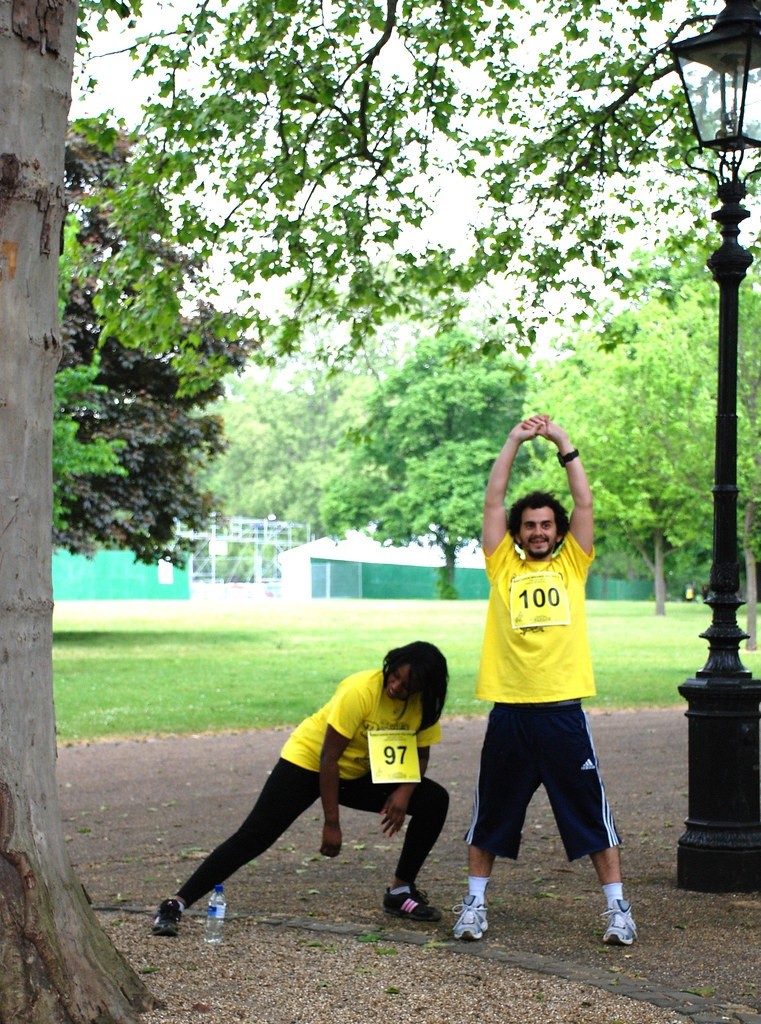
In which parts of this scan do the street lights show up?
[669,0,761,893]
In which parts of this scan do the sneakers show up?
[152,897,183,935]
[383,883,441,921]
[600,899,638,946]
[452,895,488,940]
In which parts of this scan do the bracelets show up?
[556,448,579,468]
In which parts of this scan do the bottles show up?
[204,884,227,942]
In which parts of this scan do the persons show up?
[150,639,454,936]
[449,411,640,948]
